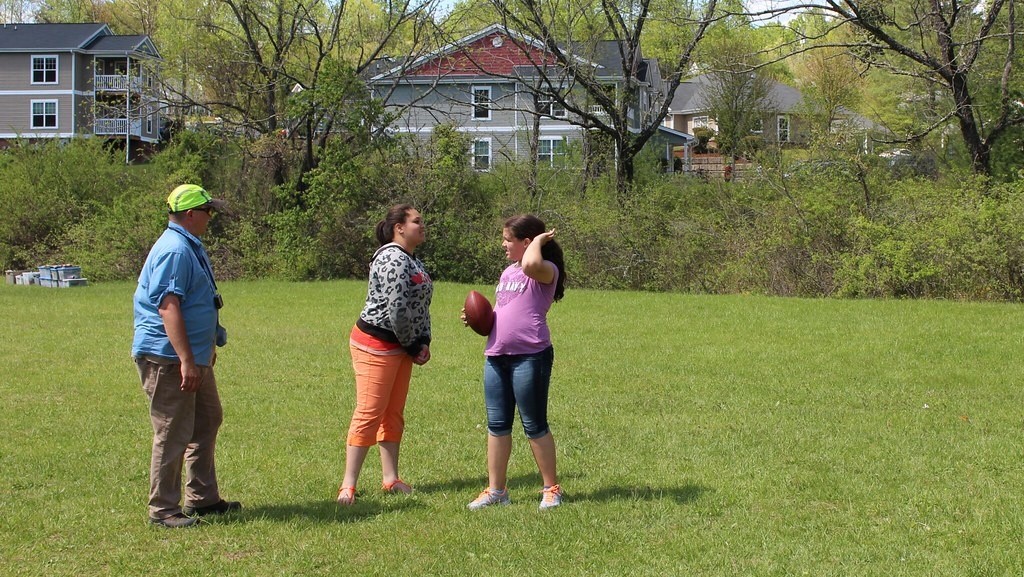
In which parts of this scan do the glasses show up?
[192,205,215,216]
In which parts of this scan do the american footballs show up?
[464,290,493,335]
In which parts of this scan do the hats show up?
[167,183,224,214]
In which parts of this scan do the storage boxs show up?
[21,271,39,285]
[38,276,51,288]
[31,271,40,285]
[49,267,59,280]
[14,274,24,284]
[50,279,58,287]
[57,277,87,287]
[4,269,30,285]
[56,267,80,279]
[37,264,61,279]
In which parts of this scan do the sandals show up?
[336,484,357,506]
[381,477,417,495]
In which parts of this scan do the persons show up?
[460,215,566,510]
[131,183,240,528]
[334,204,433,507]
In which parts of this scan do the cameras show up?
[215,293,223,309]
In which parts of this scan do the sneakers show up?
[148,511,200,528]
[465,485,510,512]
[537,482,563,509]
[183,498,242,517]
